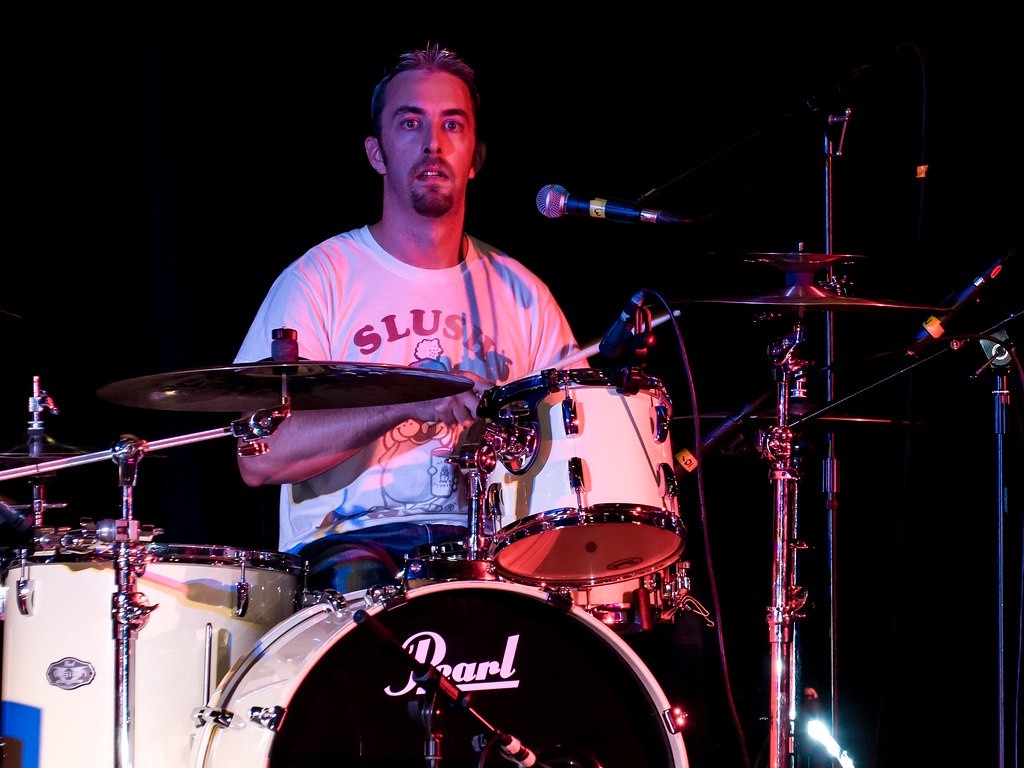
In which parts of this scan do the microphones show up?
[536,185,681,225]
[499,731,550,768]
[906,259,1005,356]
[673,392,778,481]
[599,289,645,358]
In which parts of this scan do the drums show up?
[191,578,691,768]
[482,366,691,590]
[403,533,677,645]
[0,534,310,768]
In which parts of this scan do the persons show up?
[228,46,593,591]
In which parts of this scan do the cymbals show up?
[669,394,927,428]
[91,353,476,414]
[647,249,959,315]
